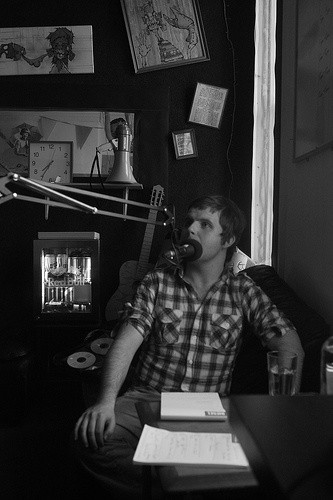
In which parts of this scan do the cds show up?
[66,352,96,369]
[90,338,114,355]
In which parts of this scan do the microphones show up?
[164,244,196,259]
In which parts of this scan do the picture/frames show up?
[0,25,94,76]
[115,0,211,76]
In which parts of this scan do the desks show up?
[134,394,333,500]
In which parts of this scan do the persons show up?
[73,194,305,500]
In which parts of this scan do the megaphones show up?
[95,125,138,185]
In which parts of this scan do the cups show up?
[268,351,297,396]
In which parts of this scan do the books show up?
[132,424,249,469]
[160,392,227,422]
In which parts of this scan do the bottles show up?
[321,336,333,397]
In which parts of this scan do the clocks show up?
[29,141,73,183]
